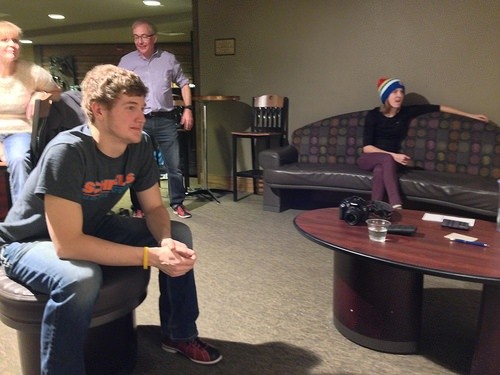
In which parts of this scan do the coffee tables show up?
[293,207,500,375]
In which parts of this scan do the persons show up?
[117,20,194,218]
[0,21,62,205]
[0,64,223,375]
[357,79,488,209]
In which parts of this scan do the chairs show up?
[229,95,287,201]
[0,93,52,205]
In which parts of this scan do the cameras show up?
[340,197,372,226]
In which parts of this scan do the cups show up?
[365,218,391,242]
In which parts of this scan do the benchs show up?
[256,109,500,223]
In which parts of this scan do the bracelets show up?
[184,105,193,110]
[143,247,149,269]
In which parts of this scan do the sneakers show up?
[173,204,192,218]
[132,209,143,219]
[161,335,223,364]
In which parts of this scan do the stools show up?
[0,261,151,375]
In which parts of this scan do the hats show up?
[377,77,404,104]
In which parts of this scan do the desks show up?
[168,95,241,204]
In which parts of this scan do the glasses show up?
[133,33,155,41]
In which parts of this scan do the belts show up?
[144,111,174,119]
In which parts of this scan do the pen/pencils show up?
[450,238,487,247]
